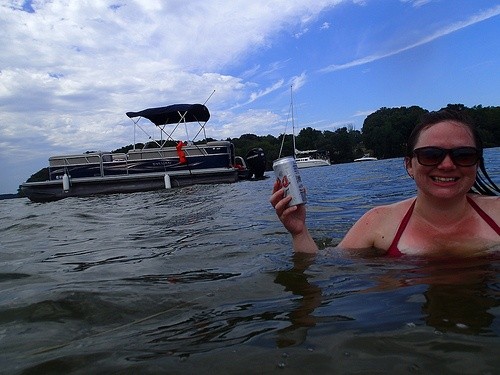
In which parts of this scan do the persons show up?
[269,115,500,258]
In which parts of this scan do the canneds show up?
[272,156,307,207]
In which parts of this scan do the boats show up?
[354,153,378,161]
[279,85,331,167]
[20,102,265,202]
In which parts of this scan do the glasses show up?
[410,146,481,167]
